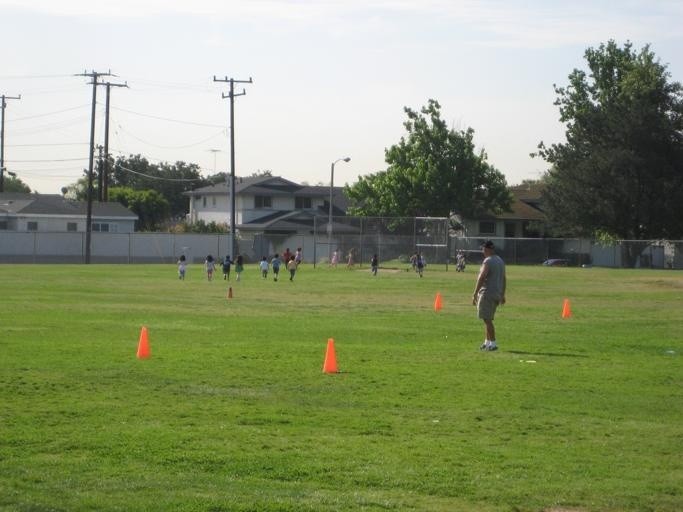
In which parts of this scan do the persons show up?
[218,254,233,281]
[232,256,244,281]
[176,255,186,280]
[203,255,216,281]
[294,247,304,265]
[286,256,296,281]
[269,252,281,282]
[259,256,268,278]
[330,247,466,279]
[282,248,291,269]
[471,239,507,351]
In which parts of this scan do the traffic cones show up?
[226,286,234,299]
[433,289,444,311]
[319,336,342,374]
[561,297,573,320]
[132,325,155,361]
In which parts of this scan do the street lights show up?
[327,156,353,262]
[210,148,221,174]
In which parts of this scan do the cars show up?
[540,256,576,267]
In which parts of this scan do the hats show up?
[480,240,494,247]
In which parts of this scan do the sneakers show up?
[478,343,498,351]
